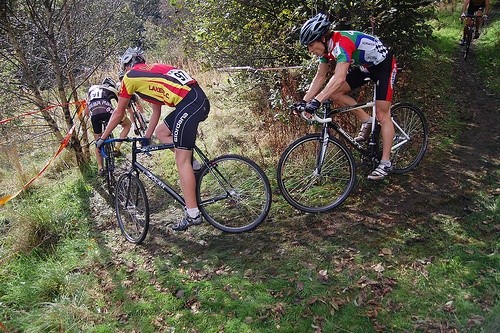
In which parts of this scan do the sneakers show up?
[97,167,105,176]
[112,149,122,157]
[474,31,480,39]
[459,37,466,46]
[192,166,210,176]
[367,161,394,182]
[171,211,204,231]
[354,117,379,143]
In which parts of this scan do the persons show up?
[460,0,490,45]
[289,13,399,179]
[88,46,211,231]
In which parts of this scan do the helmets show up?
[102,77,116,88]
[120,46,148,71]
[118,70,126,80]
[299,13,331,48]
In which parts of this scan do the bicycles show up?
[128,98,148,137]
[100,137,272,244]
[277,76,428,213]
[100,120,116,188]
[461,14,485,60]
